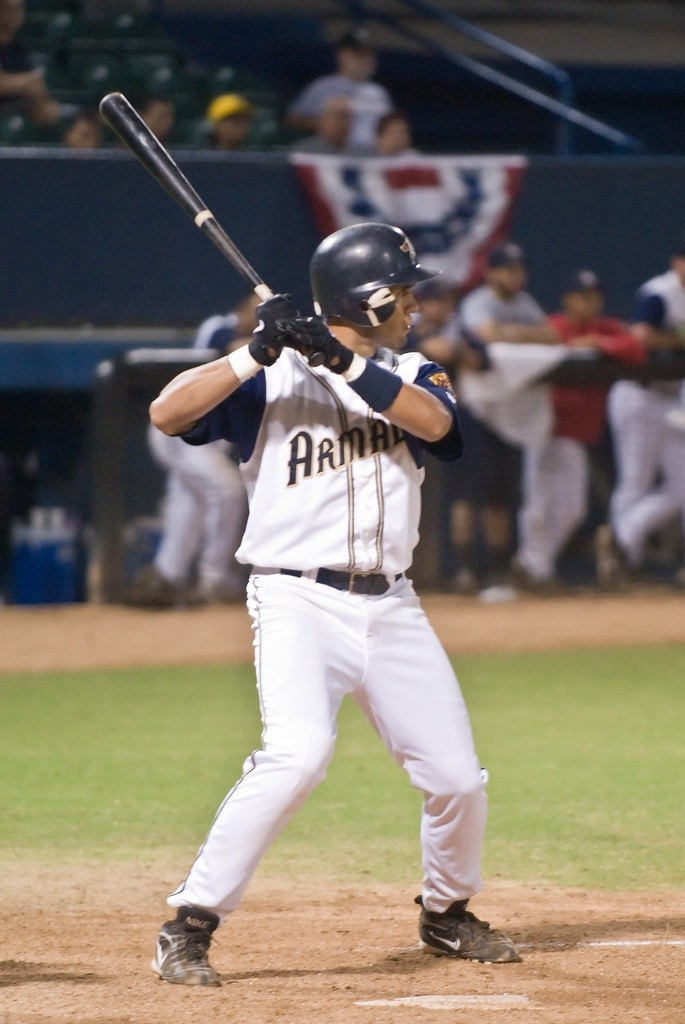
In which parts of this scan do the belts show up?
[282,568,402,594]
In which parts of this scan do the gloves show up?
[248,293,298,367]
[274,316,354,374]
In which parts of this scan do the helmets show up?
[309,221,441,327]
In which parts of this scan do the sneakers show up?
[415,893,521,964]
[151,906,220,987]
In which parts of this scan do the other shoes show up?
[595,525,630,594]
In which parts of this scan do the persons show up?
[149,221,523,987]
[448,240,685,593]
[140,91,177,138]
[60,112,96,149]
[0,0,60,122]
[150,288,265,603]
[403,280,491,381]
[285,33,417,156]
[208,94,253,151]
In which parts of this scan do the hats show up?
[565,272,598,294]
[486,242,524,269]
[208,94,252,122]
[340,26,376,59]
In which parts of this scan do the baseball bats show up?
[98,91,327,368]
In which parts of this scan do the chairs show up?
[0,0,314,152]
